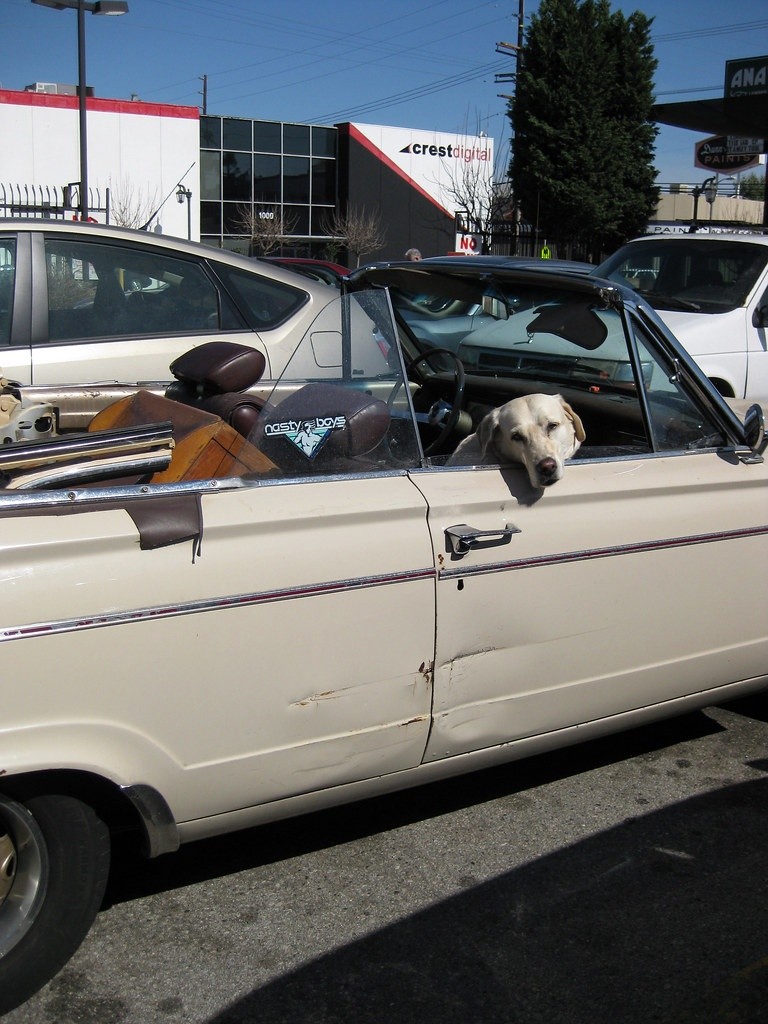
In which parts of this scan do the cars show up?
[256,254,599,300]
[0,256,768,1016]
[457,233,768,401]
[0,219,497,387]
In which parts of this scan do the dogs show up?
[445,392,586,489]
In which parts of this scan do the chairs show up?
[261,383,395,478]
[165,341,274,438]
[668,270,727,294]
[81,271,130,338]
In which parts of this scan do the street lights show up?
[689,177,716,235]
[31,0,129,285]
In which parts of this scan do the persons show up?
[405,248,422,261]
